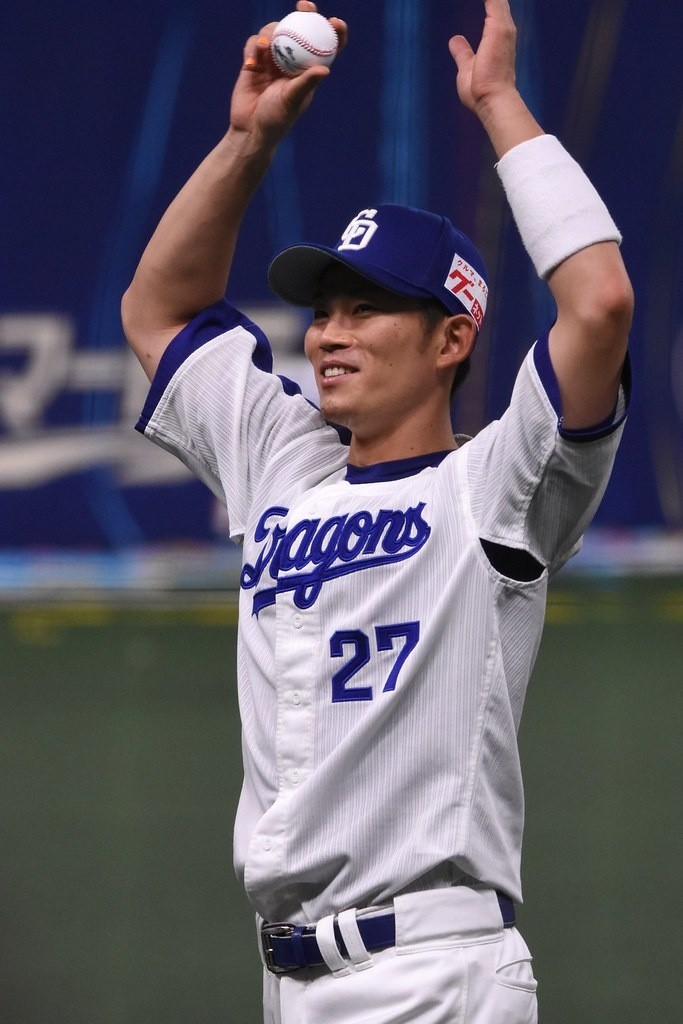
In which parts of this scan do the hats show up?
[268,202,491,339]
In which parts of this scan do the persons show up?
[118,0,636,1024]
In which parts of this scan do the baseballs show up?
[266,7,342,79]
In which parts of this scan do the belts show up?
[258,887,517,973]
